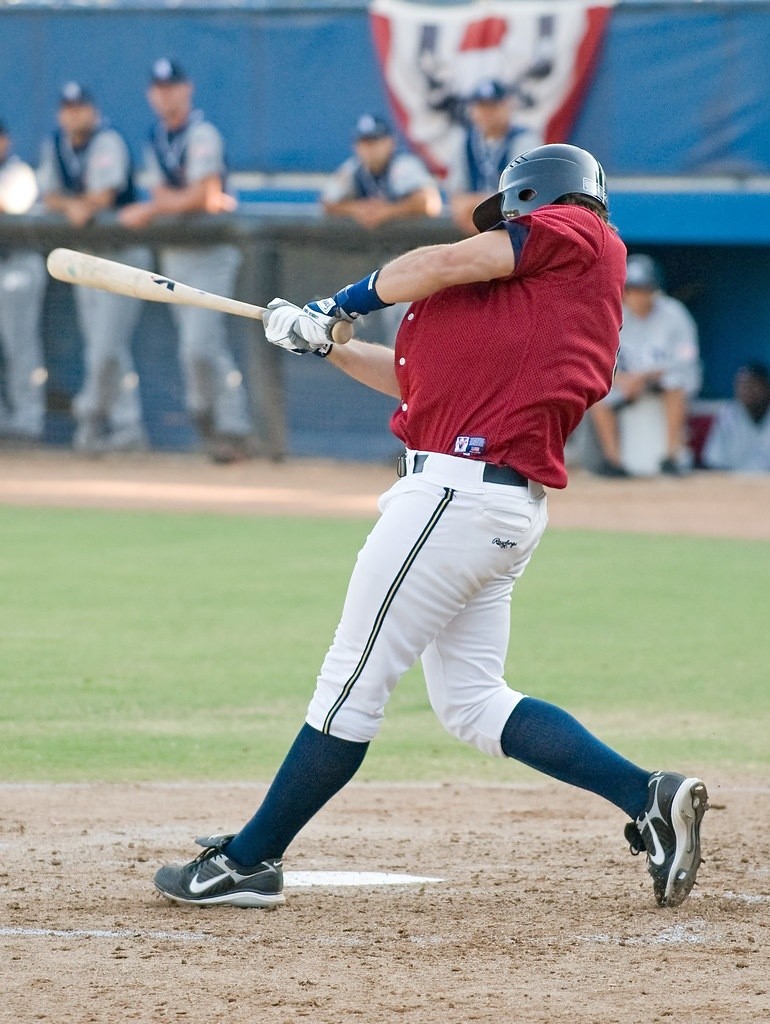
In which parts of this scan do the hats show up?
[57,83,88,106]
[148,60,182,83]
[353,115,388,141]
[621,255,658,286]
[471,81,504,103]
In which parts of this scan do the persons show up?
[118,53,250,459]
[156,143,710,908]
[320,114,442,338]
[591,251,706,478]
[33,80,154,456]
[701,363,770,469]
[0,122,50,445]
[443,82,546,236]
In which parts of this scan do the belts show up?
[397,453,529,487]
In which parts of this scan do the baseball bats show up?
[45,247,356,345]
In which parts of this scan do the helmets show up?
[471,143,610,234]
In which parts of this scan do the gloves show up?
[261,295,337,358]
[304,282,360,328]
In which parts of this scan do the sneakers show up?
[154,833,287,909]
[625,771,709,910]
